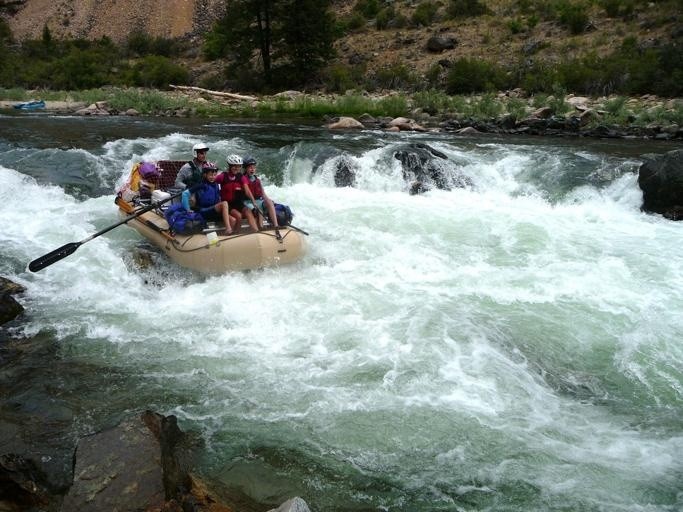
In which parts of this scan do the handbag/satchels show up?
[275,204,292,225]
[165,203,208,235]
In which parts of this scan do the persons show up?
[181,162,237,236]
[240,158,278,231]
[215,153,259,234]
[175,143,216,209]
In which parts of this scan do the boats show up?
[13,101,45,111]
[114,188,310,274]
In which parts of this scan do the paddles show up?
[29,190,181,272]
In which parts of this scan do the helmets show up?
[192,144,209,158]
[203,162,218,171]
[243,157,256,165]
[227,155,243,165]
